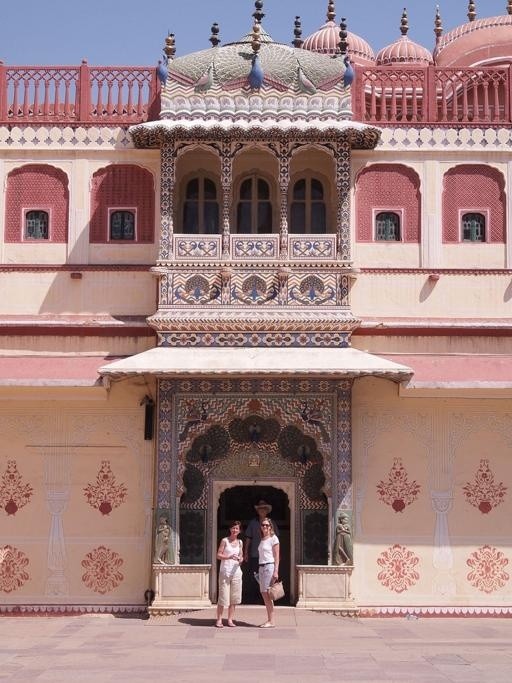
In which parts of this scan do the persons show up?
[256,517,282,628]
[241,499,281,603]
[214,520,244,628]
[155,518,172,564]
[336,512,353,566]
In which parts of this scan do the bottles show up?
[254,572,261,584]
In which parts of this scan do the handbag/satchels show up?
[268,581,285,601]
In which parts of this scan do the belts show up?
[259,562,275,566]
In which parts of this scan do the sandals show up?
[216,623,237,627]
[260,625,276,628]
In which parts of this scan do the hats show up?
[254,500,273,513]
